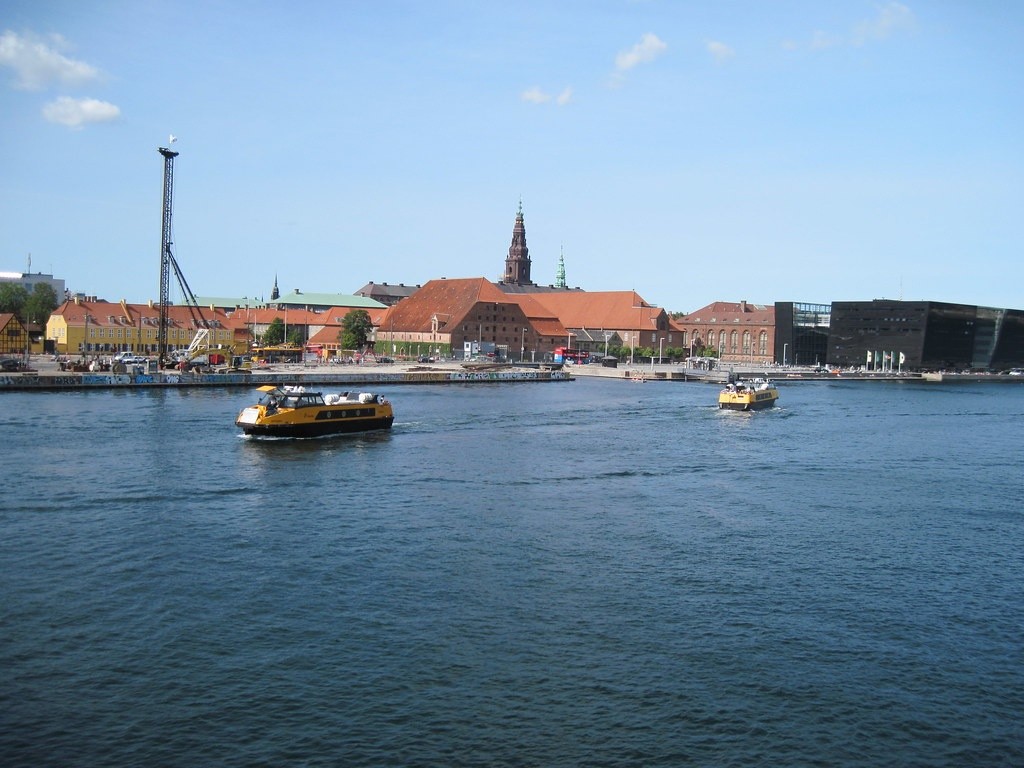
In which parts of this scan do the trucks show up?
[113,352,150,363]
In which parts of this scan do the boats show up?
[719,378,779,411]
[235,386,395,437]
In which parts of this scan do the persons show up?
[727,382,734,392]
[736,380,744,386]
[348,355,352,365]
[356,352,361,363]
[749,379,755,393]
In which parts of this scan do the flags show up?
[892,352,896,363]
[876,351,880,362]
[900,352,905,364]
[868,351,872,362]
[883,352,888,362]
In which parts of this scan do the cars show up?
[0,359,21,371]
[1009,368,1024,376]
[463,356,476,361]
[470,357,487,362]
[123,356,144,364]
[493,357,506,363]
[487,351,496,356]
[574,358,589,364]
[417,356,435,364]
[379,358,394,363]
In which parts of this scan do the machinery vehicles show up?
[184,346,252,374]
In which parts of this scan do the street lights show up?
[25,311,36,365]
[242,297,249,354]
[719,341,723,359]
[521,328,527,362]
[783,344,788,366]
[631,337,637,363]
[691,340,693,357]
[391,302,396,359]
[605,335,610,357]
[83,313,87,365]
[282,302,287,343]
[568,333,573,349]
[660,338,665,364]
[751,342,755,364]
